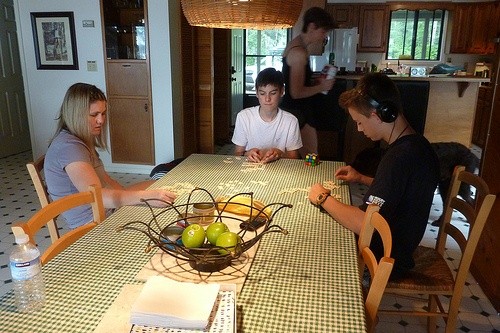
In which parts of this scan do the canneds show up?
[161,202,215,249]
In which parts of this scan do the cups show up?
[356,66,361,72]
[340,67,346,72]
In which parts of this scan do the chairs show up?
[25,153,63,245]
[358,204,394,333]
[11,184,106,268]
[363,164,496,333]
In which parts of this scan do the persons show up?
[309,71,440,263]
[44,83,176,230]
[232,68,303,164]
[283,7,336,158]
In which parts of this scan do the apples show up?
[205,222,230,244]
[216,232,242,255]
[182,224,205,248]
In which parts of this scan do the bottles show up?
[104,0,144,25]
[8,233,46,313]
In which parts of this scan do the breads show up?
[217,197,272,216]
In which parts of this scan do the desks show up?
[0,154,369,333]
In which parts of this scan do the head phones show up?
[358,90,399,123]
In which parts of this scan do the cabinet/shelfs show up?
[445,2,500,55]
[325,2,390,53]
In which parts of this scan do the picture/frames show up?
[30,11,79,71]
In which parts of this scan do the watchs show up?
[315,192,330,207]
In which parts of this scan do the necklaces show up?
[396,123,411,140]
